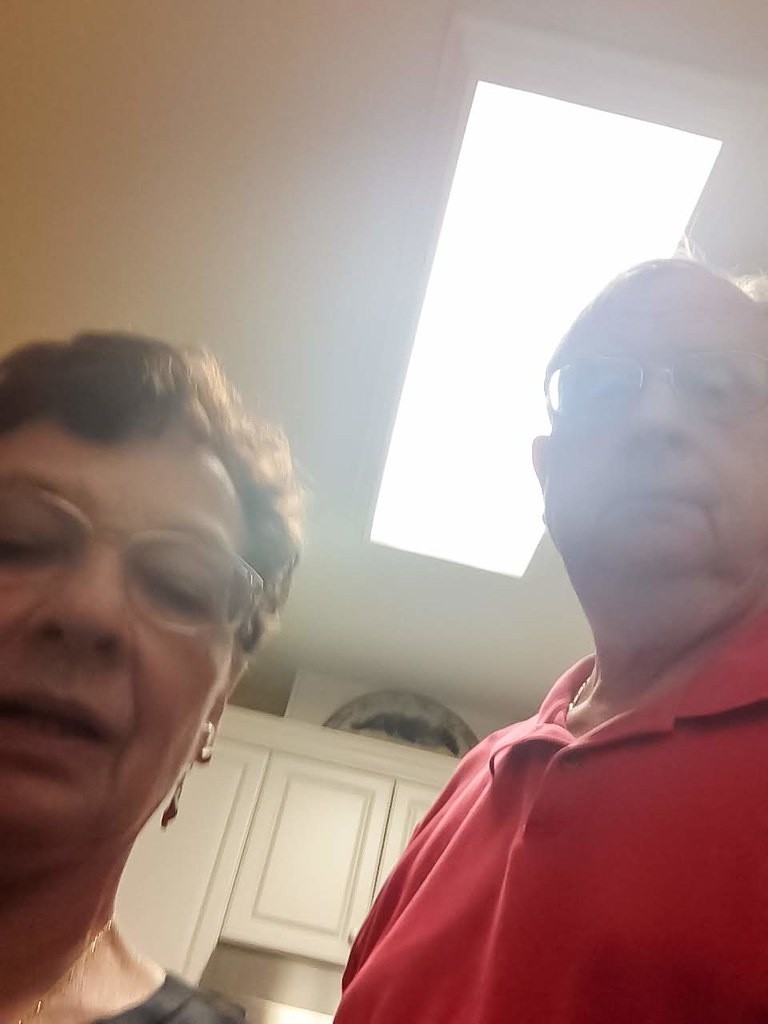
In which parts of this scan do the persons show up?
[0,329,302,1024]
[332,260,768,1024]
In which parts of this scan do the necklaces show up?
[18,911,117,1024]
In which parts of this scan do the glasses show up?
[540,352,768,427]
[0,478,263,640]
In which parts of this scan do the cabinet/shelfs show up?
[117,697,472,1024]
